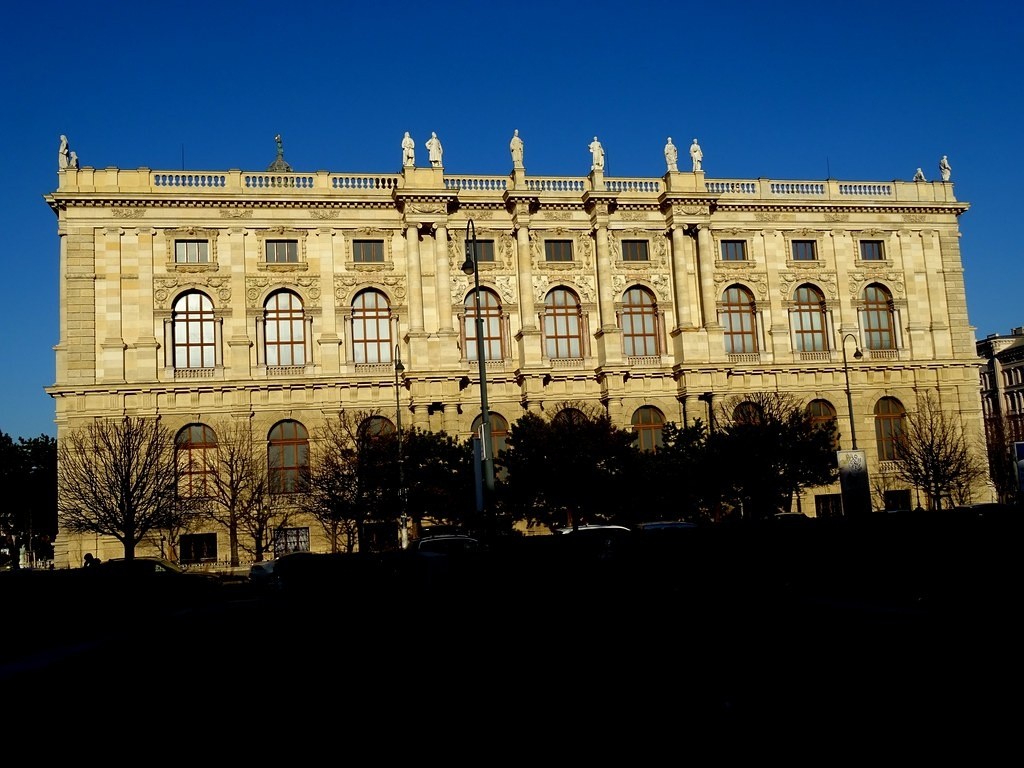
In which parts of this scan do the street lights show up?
[842,334,862,450]
[462,219,494,490]
[395,344,408,549]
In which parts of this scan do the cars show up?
[91,509,909,623]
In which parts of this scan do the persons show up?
[84,553,101,569]
